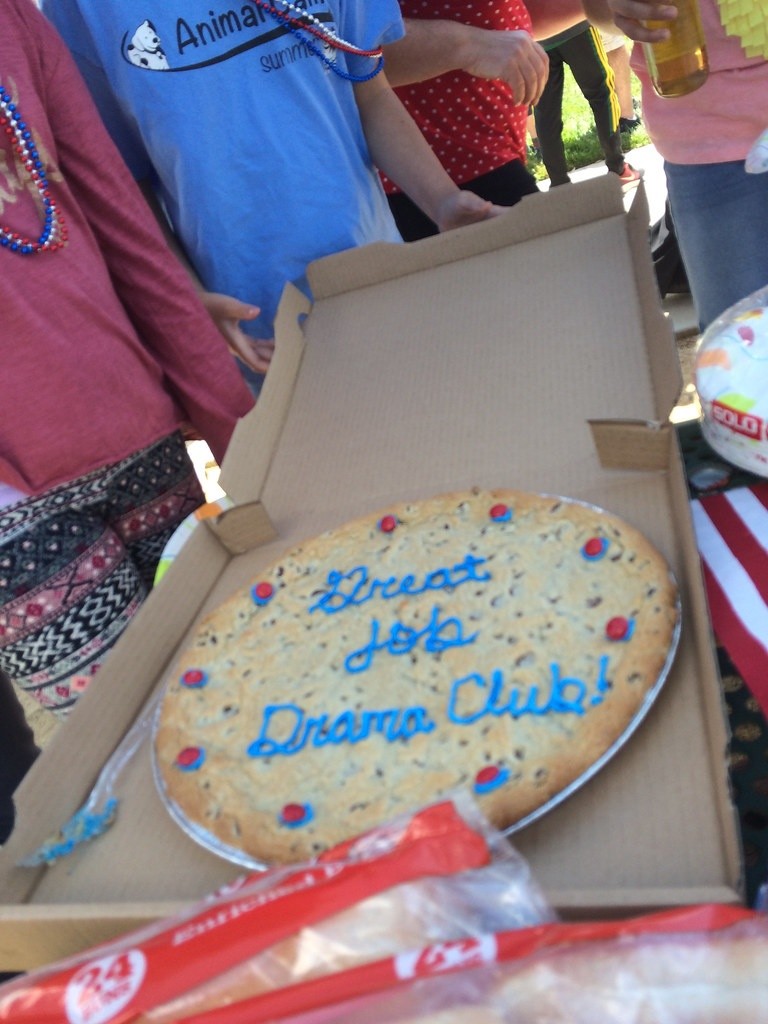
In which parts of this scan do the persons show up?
[0,0,258,726]
[377,0,549,243]
[522,0,645,192]
[607,0,768,337]
[41,0,514,404]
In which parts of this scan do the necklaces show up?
[0,85,69,254]
[255,0,384,83]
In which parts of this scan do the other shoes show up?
[615,116,642,133]
[533,146,544,161]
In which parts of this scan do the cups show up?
[639,0,710,99]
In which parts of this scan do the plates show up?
[147,502,683,877]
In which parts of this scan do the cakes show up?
[152,485,678,870]
[697,301,768,480]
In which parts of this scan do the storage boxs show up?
[0,167,752,978]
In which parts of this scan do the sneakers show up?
[619,161,646,193]
